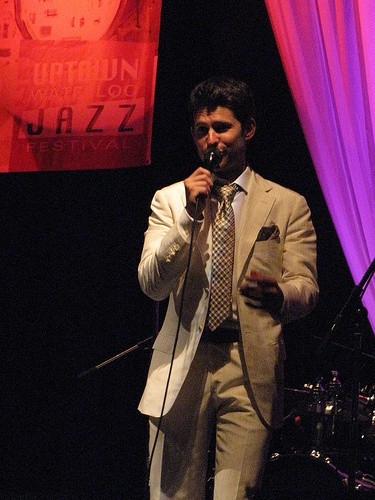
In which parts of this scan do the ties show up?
[205,184,237,331]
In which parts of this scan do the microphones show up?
[198,149,219,201]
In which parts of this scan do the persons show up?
[137,77,317,498]
